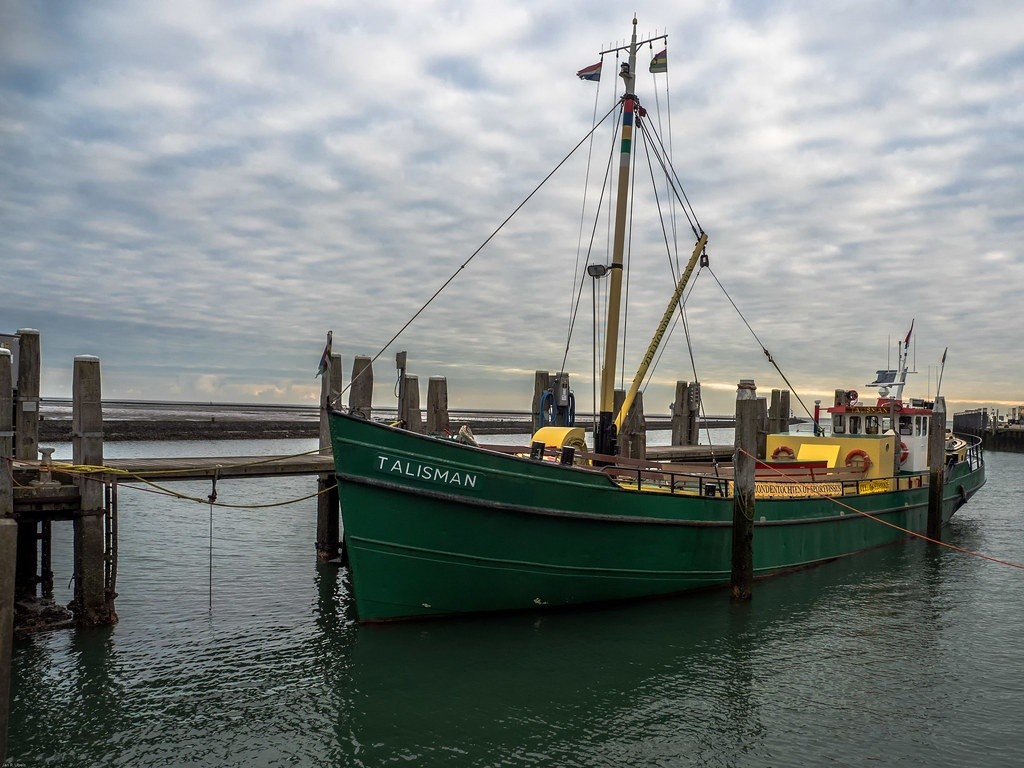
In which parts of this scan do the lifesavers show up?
[900,441,908,462]
[845,449,871,473]
[772,446,794,459]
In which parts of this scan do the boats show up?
[320,13,985,625]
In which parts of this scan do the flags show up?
[576,62,602,81]
[315,331,334,379]
[942,347,948,363]
[650,49,667,72]
[904,319,914,346]
